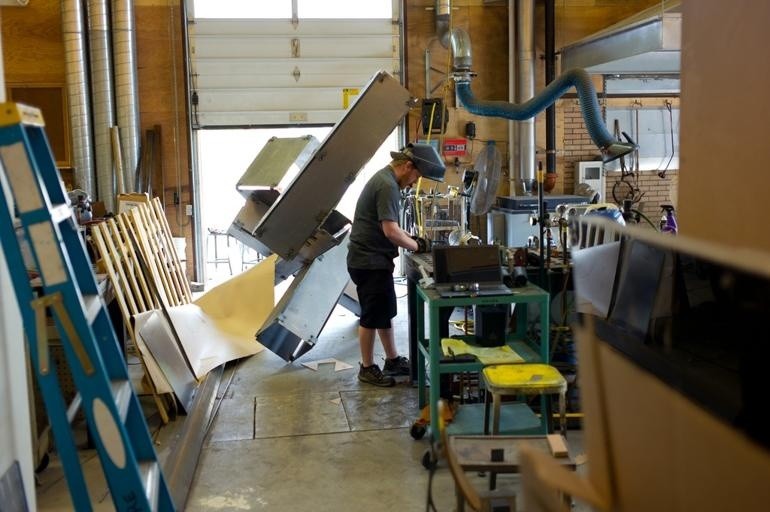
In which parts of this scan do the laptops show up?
[432,245,514,299]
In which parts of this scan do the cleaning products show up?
[660,203,678,234]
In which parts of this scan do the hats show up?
[389,146,417,162]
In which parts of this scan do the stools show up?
[481,362,569,493]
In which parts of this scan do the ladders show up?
[0,102,176,512]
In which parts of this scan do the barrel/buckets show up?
[473,304,507,346]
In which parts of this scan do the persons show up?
[345,147,432,388]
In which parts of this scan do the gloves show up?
[410,235,433,254]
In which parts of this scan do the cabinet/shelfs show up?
[413,283,550,464]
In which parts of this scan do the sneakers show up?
[381,357,413,377]
[358,361,397,389]
